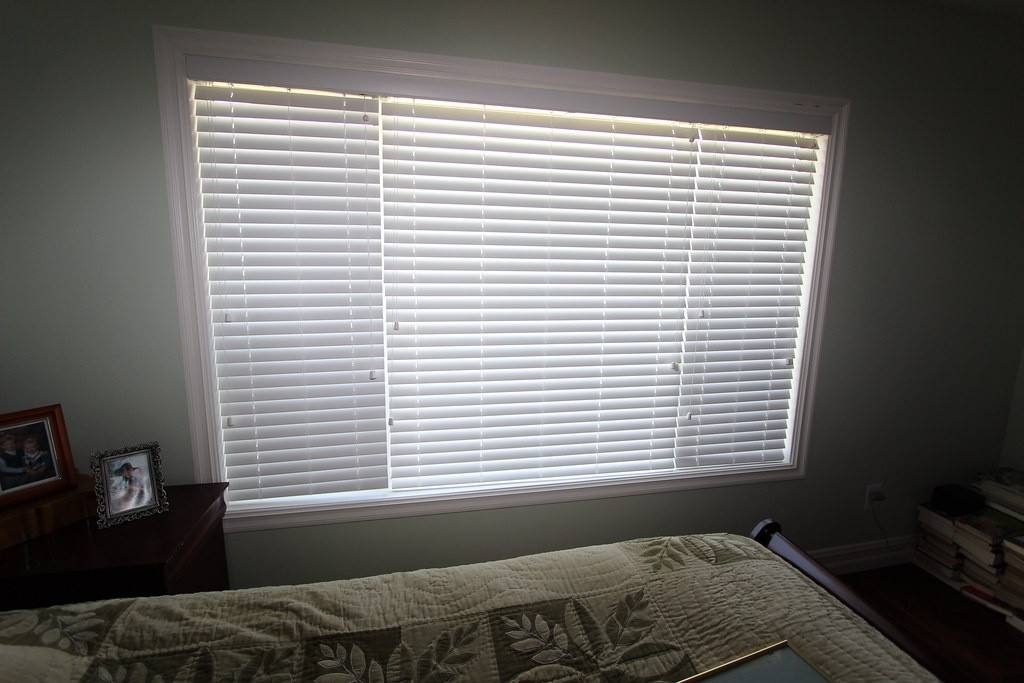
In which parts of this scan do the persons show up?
[110,463,146,514]
[0,436,52,491]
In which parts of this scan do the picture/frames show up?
[89,441,171,530]
[0,403,79,511]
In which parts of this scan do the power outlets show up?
[864,484,881,512]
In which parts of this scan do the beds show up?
[0,518,955,683]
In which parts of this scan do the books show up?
[914,466,1024,635]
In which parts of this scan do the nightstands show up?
[0,482,228,612]
